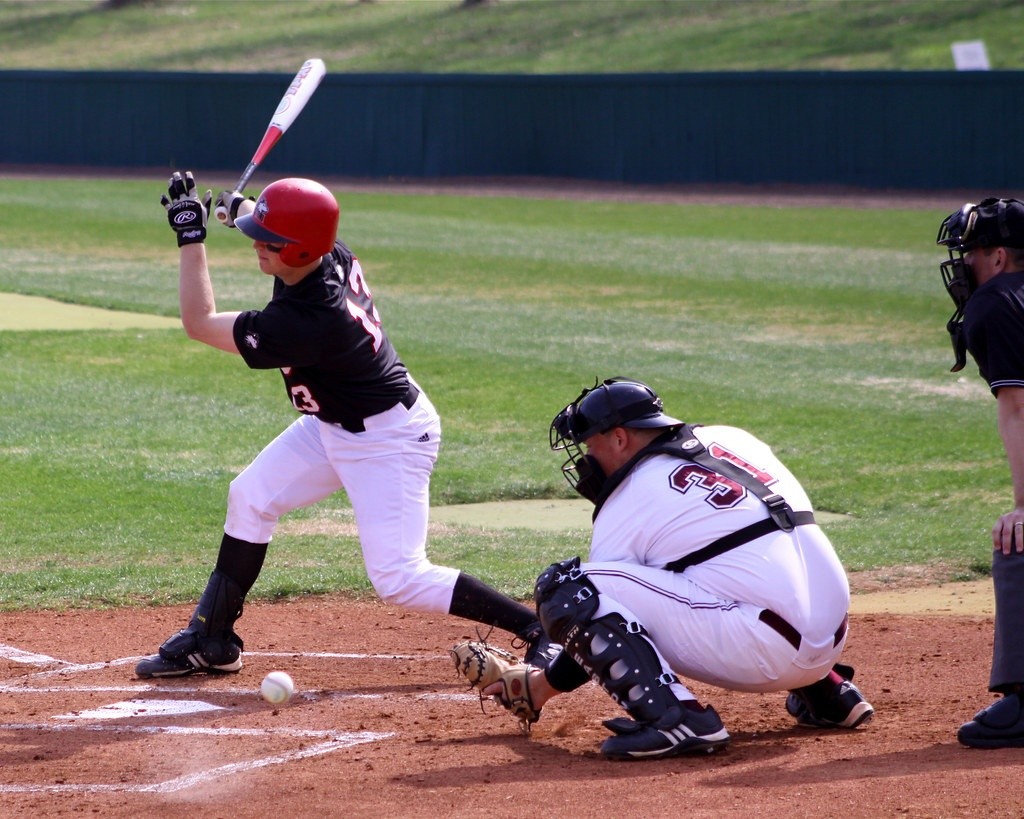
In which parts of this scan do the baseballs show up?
[261,669,296,708]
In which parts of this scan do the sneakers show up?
[136,629,243,680]
[600,703,729,762]
[786,677,874,729]
[511,617,564,669]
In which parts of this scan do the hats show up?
[555,375,685,441]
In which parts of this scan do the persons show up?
[935,196,1024,748]
[453,378,874,761]
[138,170,562,679]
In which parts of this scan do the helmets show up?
[936,191,1024,249]
[234,177,341,267]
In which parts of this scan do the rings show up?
[1014,520,1024,528]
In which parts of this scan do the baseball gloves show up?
[447,621,544,728]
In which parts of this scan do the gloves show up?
[213,189,254,226]
[160,171,208,244]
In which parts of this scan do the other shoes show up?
[958,694,1024,748]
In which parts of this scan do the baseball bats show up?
[211,58,326,225]
